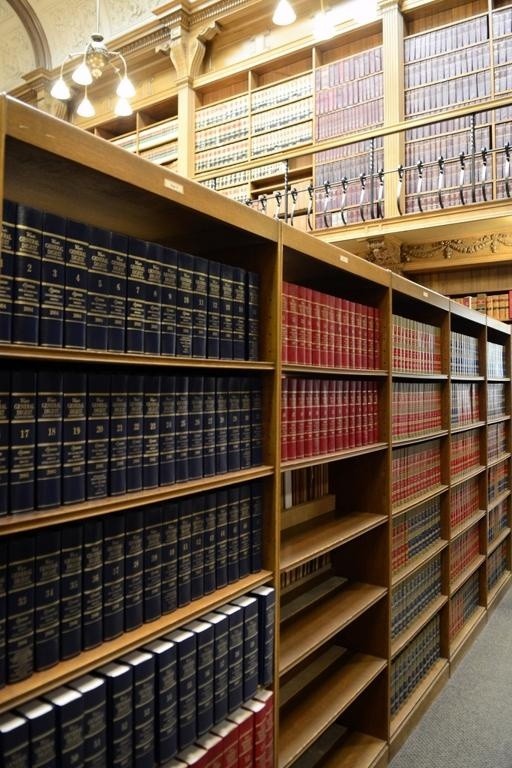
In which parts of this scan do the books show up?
[1,199,274,767]
[115,7,512,229]
[392,314,511,715]
[282,284,385,587]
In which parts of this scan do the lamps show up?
[52,1,138,119]
